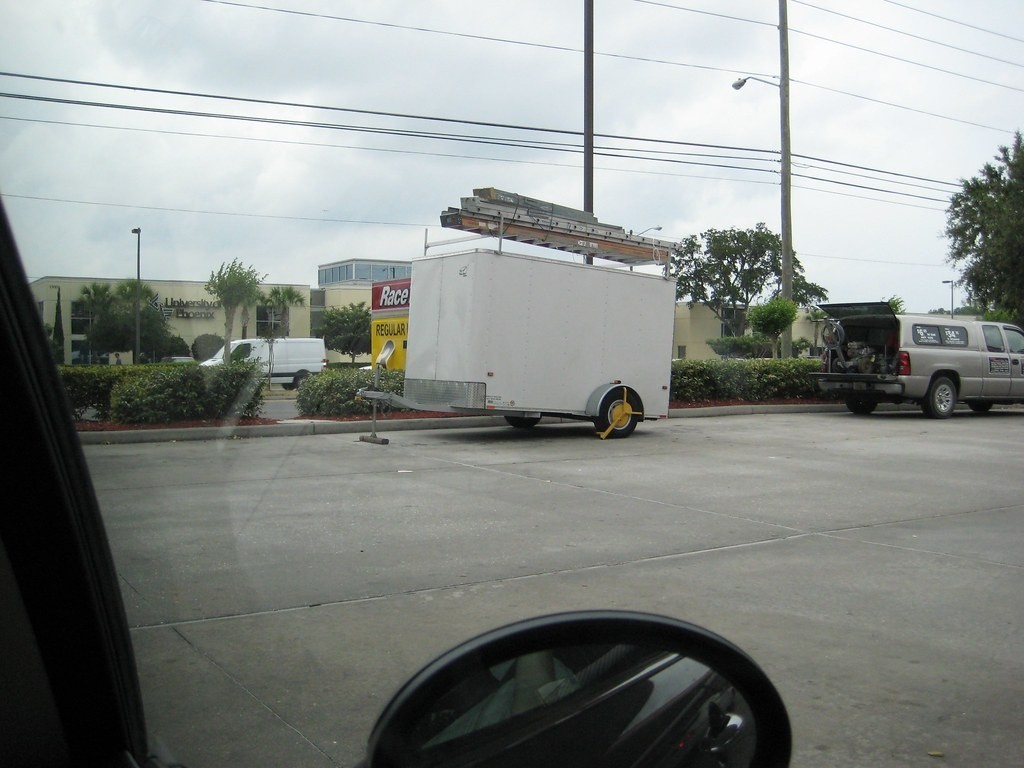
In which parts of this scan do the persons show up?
[114,353,121,365]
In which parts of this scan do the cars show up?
[159,357,195,365]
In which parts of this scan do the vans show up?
[202,338,326,383]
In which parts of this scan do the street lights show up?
[941,280,955,319]
[629,225,663,272]
[733,77,794,362]
[131,227,141,366]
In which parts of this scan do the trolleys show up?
[810,315,896,375]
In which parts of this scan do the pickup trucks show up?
[808,300,1024,422]
